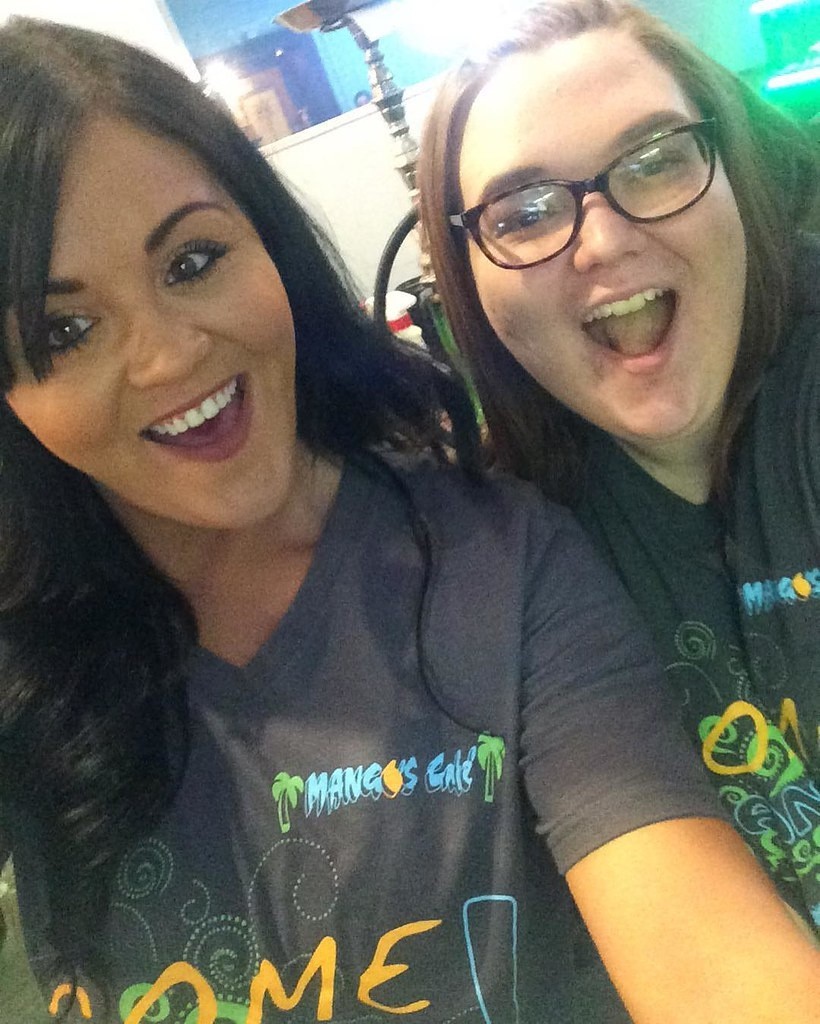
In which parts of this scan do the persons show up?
[0,13,820,1024]
[417,0,820,946]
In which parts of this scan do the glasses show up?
[447,117,717,271]
